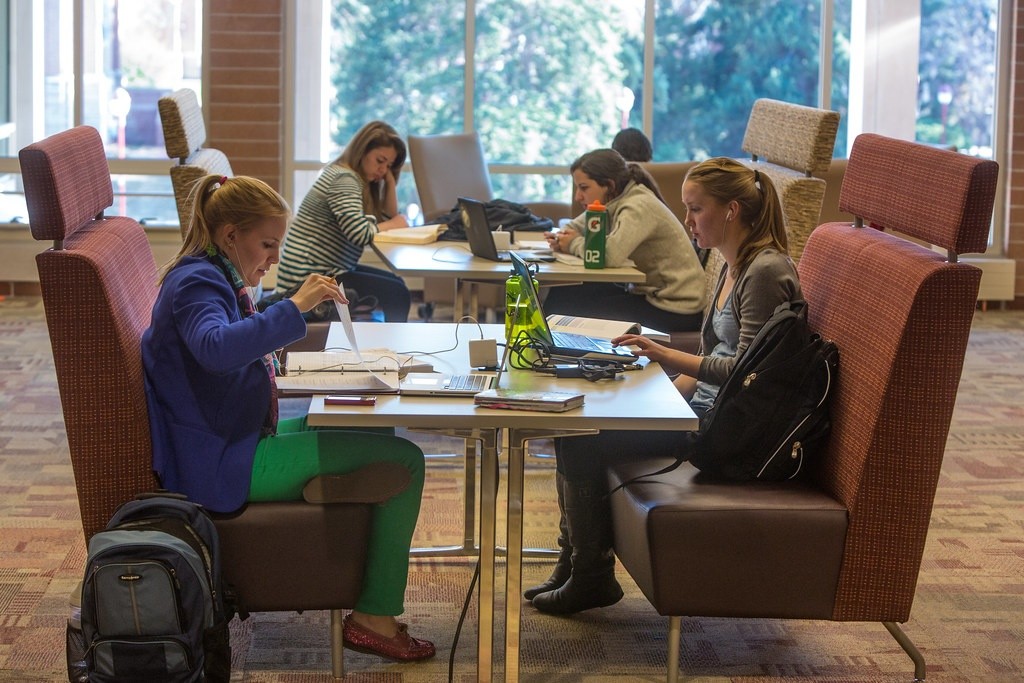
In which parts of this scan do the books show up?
[545,314,672,344]
[275,352,434,396]
[474,388,584,412]
[373,224,449,244]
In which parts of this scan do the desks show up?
[369,227,646,322]
[307,322,699,683]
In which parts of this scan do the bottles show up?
[505,269,539,345]
[584,200,606,268]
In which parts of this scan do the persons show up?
[275,121,411,323]
[612,128,652,162]
[144,174,436,661]
[524,158,809,614]
[543,148,708,333]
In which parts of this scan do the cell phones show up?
[324,395,376,405]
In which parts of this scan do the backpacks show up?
[64,489,232,683]
[688,299,840,487]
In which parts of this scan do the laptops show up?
[509,251,639,363]
[457,195,557,262]
[400,293,520,396]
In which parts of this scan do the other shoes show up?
[342,616,436,662]
[303,462,411,505]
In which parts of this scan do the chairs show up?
[605,133,1000,683]
[408,131,494,225]
[158,88,235,243]
[700,98,840,316]
[18,125,413,683]
[572,162,701,240]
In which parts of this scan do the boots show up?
[527,466,624,617]
